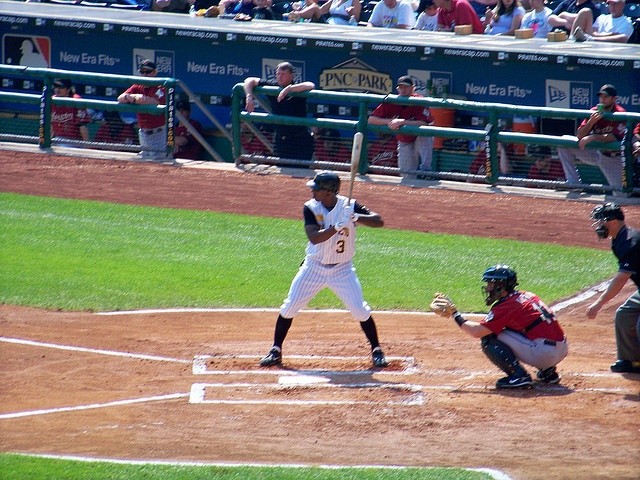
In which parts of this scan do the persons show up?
[548,0,599,38]
[527,145,567,188]
[631,123,640,158]
[291,0,318,23]
[366,0,414,30]
[94,111,138,153]
[368,75,435,180]
[259,170,387,367]
[519,0,553,37]
[289,0,362,26]
[414,0,439,31]
[203,0,273,19]
[468,0,497,18]
[584,201,640,373]
[117,59,167,156]
[429,265,569,388]
[557,84,627,197]
[173,99,202,160]
[313,128,351,171]
[433,0,484,34]
[243,62,315,169]
[482,0,526,36]
[367,132,399,176]
[569,0,634,44]
[465,141,514,186]
[51,79,91,148]
[230,122,272,165]
[140,0,198,12]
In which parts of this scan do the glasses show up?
[55,84,66,88]
[533,155,546,161]
[139,69,152,74]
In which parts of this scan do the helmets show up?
[482,266,518,306]
[590,201,626,240]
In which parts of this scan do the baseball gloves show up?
[429,293,458,318]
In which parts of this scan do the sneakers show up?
[496,373,532,388]
[259,350,282,367]
[572,26,590,41]
[537,369,560,386]
[283,11,302,23]
[610,361,636,372]
[372,350,385,368]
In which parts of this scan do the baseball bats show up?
[347,132,363,206]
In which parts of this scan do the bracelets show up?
[245,97,254,104]
[125,94,129,101]
[452,310,468,328]
[245,93,253,98]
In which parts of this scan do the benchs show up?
[434,147,614,195]
[0,117,234,165]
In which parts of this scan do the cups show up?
[597,103,604,113]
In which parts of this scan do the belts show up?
[139,127,163,136]
[600,150,621,157]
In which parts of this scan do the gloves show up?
[334,219,351,235]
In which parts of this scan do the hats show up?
[137,60,156,71]
[176,101,191,111]
[51,77,72,88]
[606,0,626,3]
[596,84,616,98]
[305,172,341,193]
[530,144,552,158]
[398,76,414,88]
[317,129,339,141]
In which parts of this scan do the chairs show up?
[624,3,640,19]
[273,1,292,12]
[595,2,608,14]
[356,1,378,26]
